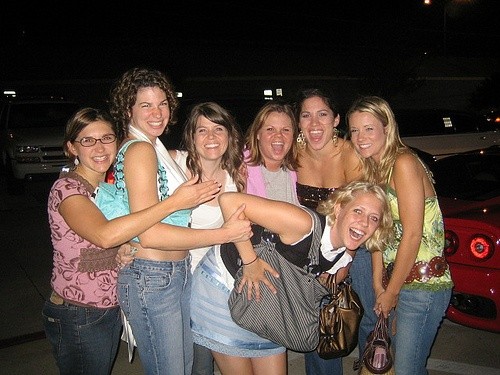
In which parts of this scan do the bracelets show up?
[243,256,258,265]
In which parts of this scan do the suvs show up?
[0,94,82,195]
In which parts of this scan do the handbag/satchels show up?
[316,271,364,360]
[356,312,396,375]
[227,206,332,355]
[94,140,191,244]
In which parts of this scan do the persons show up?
[292,87,385,375]
[114,69,254,375]
[345,96,451,375]
[168,104,240,375]
[40,107,220,375]
[188,180,388,375]
[234,105,300,207]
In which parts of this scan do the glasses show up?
[74,133,117,147]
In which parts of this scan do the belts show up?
[382,256,448,291]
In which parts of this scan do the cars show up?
[384,58,500,332]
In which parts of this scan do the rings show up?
[119,261,121,266]
